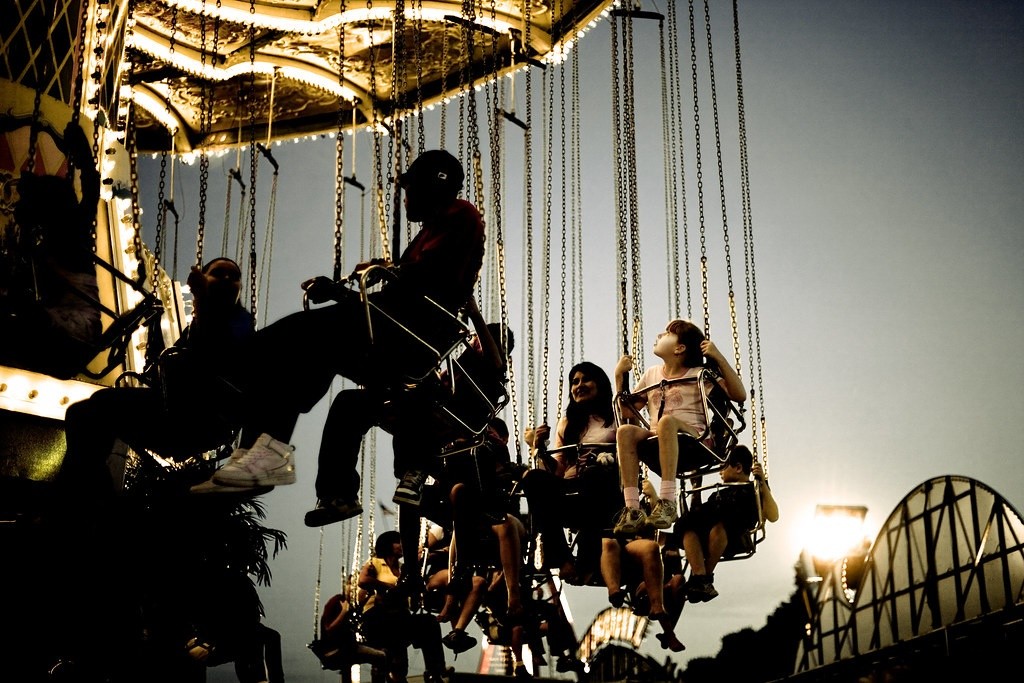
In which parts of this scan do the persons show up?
[65,258,253,465]
[305,323,514,527]
[521,362,617,579]
[613,320,746,533]
[213,150,483,487]
[321,418,584,683]
[673,446,778,603]
[600,480,685,652]
[235,622,284,683]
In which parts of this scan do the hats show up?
[397,149,465,191]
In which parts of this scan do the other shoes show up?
[443,629,477,654]
[559,562,576,580]
[534,657,547,666]
[656,633,686,652]
[442,666,454,676]
[515,665,532,677]
[556,656,585,673]
[688,572,718,603]
[574,571,594,585]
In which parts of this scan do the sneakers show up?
[644,498,678,529]
[612,506,647,534]
[213,433,296,486]
[392,470,426,507]
[189,448,275,498]
[304,496,364,527]
